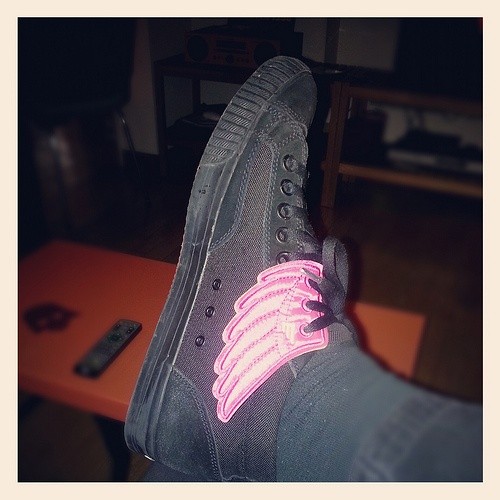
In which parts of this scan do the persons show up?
[123,53,482,482]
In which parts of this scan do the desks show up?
[18,234,428,479]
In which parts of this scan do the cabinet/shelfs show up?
[126,16,484,406]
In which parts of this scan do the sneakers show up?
[121,57,344,481]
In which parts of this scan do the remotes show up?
[73,319,143,378]
